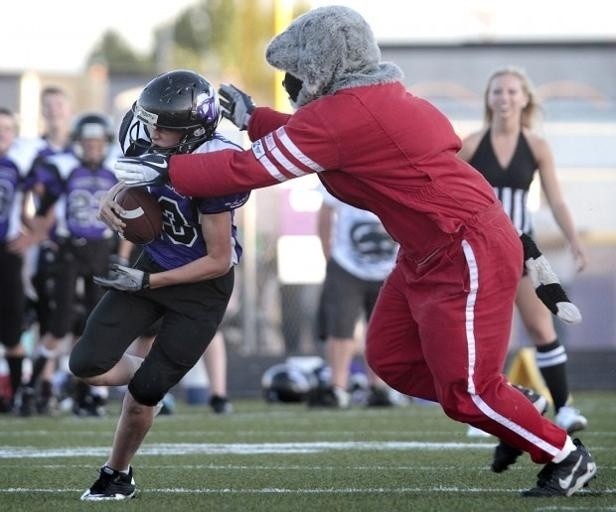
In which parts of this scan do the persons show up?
[114,5,599,499]
[453,66,590,436]
[1,87,232,416]
[308,184,409,409]
[67,68,251,503]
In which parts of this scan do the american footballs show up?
[113,187,162,244]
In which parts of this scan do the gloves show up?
[113,150,169,187]
[92,263,150,294]
[218,83,253,131]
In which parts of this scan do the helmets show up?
[129,70,220,155]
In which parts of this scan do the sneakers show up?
[80,461,136,501]
[521,438,597,497]
[491,383,548,474]
[555,406,587,434]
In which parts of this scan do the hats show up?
[264,5,404,111]
